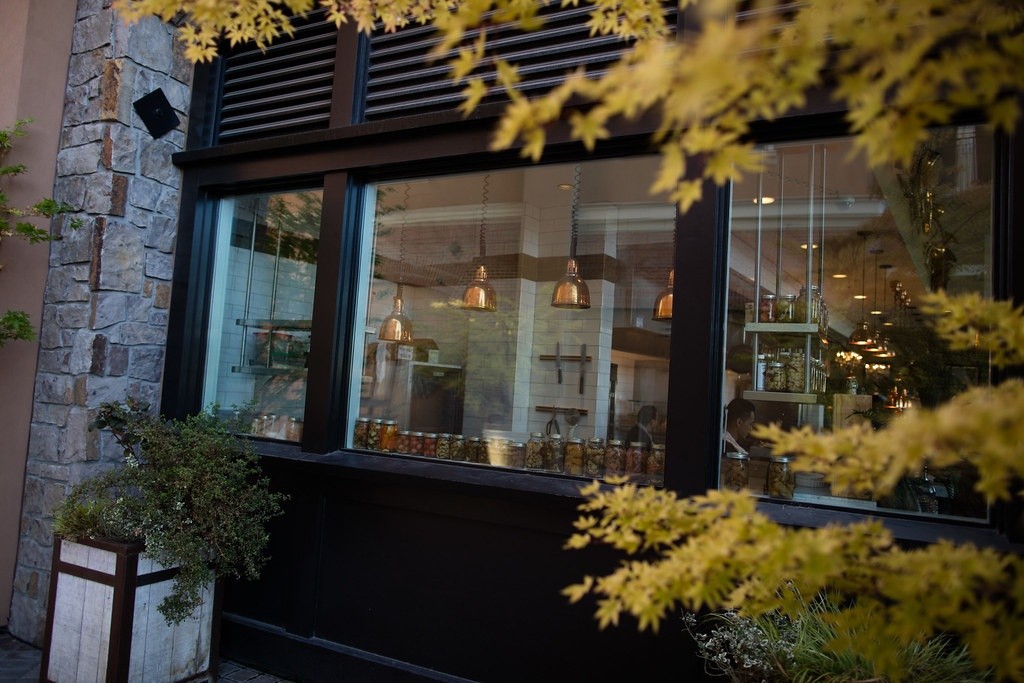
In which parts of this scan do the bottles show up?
[844,376,858,395]
[888,384,912,408]
[724,446,796,501]
[760,285,829,337]
[756,351,826,395]
[525,432,666,487]
[353,418,489,464]
[233,409,305,443]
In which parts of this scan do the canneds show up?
[251,415,304,443]
[760,283,826,392]
[725,452,796,499]
[508,432,665,487]
[351,416,491,466]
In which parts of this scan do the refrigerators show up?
[389,361,464,437]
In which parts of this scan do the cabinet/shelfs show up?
[358,356,465,435]
[232,317,376,383]
[742,321,829,402]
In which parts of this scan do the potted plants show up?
[42,397,295,683]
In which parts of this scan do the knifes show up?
[580,344,587,393]
[556,341,562,384]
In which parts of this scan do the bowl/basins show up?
[796,471,824,488]
[482,429,531,469]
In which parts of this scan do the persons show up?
[625,405,658,453]
[722,398,755,457]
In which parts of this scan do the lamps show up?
[848,231,875,345]
[551,161,592,309]
[378,182,413,343]
[652,207,678,321]
[862,249,887,352]
[884,282,900,408]
[835,351,863,373]
[873,264,896,357]
[461,173,497,313]
[895,290,907,409]
[903,298,911,408]
